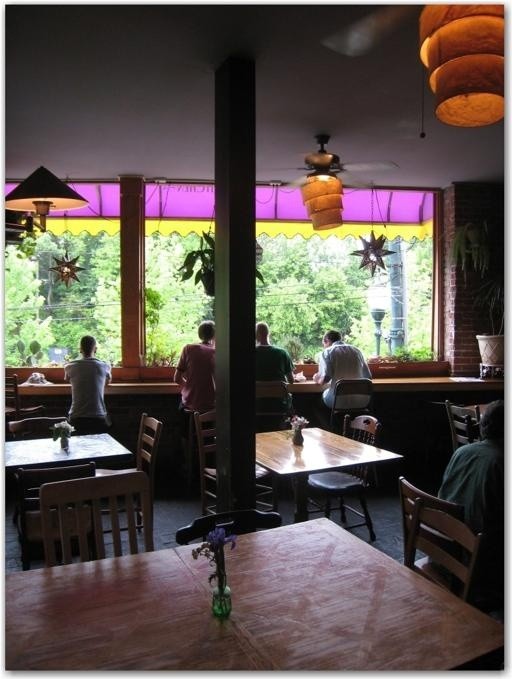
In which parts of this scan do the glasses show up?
[419,4,504,133]
[5,166,92,235]
[296,177,346,230]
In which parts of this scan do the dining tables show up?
[448,213,504,370]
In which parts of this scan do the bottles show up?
[19,373,53,386]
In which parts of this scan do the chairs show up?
[99,413,165,536]
[446,396,482,459]
[18,459,100,573]
[10,415,67,437]
[177,507,280,541]
[399,498,484,607]
[43,469,154,563]
[305,416,380,539]
[184,377,292,538]
[396,475,483,590]
[5,370,44,420]
[321,379,381,477]
[462,412,492,451]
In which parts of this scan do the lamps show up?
[43,469,154,563]
[399,498,484,607]
[18,459,100,573]
[10,415,67,437]
[177,507,280,541]
[419,4,504,133]
[5,370,44,420]
[296,177,346,230]
[5,166,92,235]
[99,413,165,536]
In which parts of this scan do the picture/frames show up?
[5,360,504,400]
[5,515,504,671]
[5,434,129,480]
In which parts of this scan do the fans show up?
[252,136,400,198]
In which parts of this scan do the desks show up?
[252,426,403,524]
[5,515,504,671]
[5,434,129,480]
[5,360,504,400]
[368,305,384,354]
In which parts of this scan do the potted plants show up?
[448,213,504,370]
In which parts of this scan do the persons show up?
[244,324,294,437]
[312,330,373,432]
[430,400,502,610]
[175,320,218,448]
[62,338,113,437]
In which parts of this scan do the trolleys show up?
[252,136,400,198]
